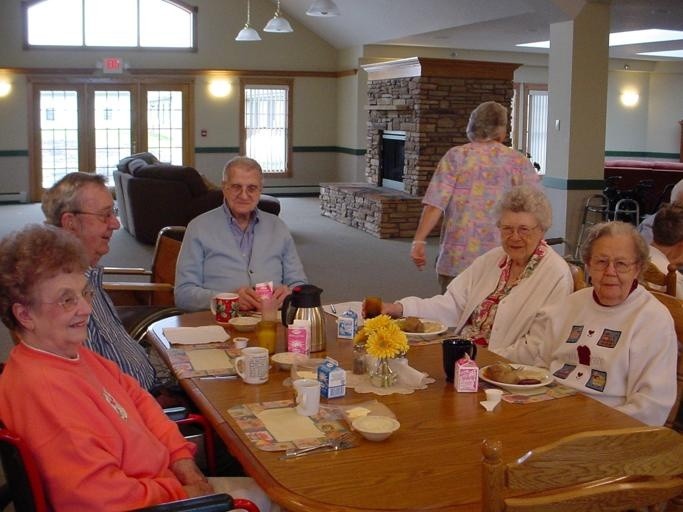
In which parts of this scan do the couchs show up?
[111,152,280,246]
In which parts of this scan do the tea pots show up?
[281,285,327,351]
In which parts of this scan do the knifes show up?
[281,443,361,461]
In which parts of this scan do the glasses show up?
[500,224,538,234]
[60,209,118,223]
[590,256,638,273]
[57,288,95,312]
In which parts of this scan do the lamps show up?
[304,0,341,17]
[233,0,262,42]
[260,0,293,33]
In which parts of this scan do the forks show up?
[287,432,352,457]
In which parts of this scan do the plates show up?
[351,415,400,442]
[479,363,555,389]
[228,316,262,332]
[271,352,309,371]
[391,318,448,339]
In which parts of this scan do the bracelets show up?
[413,240,428,245]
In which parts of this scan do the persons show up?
[361,185,575,363]
[588,179,683,299]
[409,101,540,295]
[0,221,285,512]
[534,222,678,427]
[39,171,189,408]
[173,156,310,314]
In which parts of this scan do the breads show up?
[484,361,546,384]
[400,316,424,333]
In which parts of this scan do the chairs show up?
[477,426,683,511]
[1,407,266,511]
[641,261,677,299]
[646,289,683,428]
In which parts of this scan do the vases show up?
[367,356,400,389]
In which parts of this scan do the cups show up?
[209,292,239,326]
[290,378,321,416]
[364,296,383,318]
[442,338,478,383]
[483,388,504,404]
[233,346,269,385]
[260,293,278,324]
[233,337,248,349]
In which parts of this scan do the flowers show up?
[351,314,410,363]
